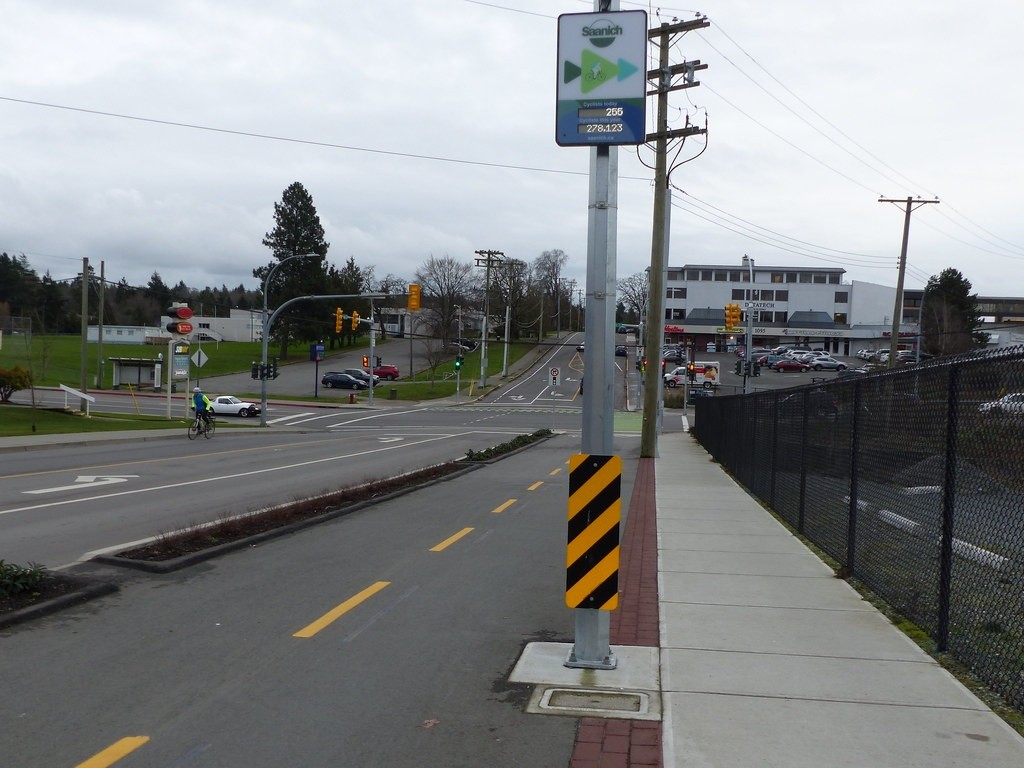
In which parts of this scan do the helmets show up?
[193,387,200,392]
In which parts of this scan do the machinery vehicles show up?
[366,356,399,380]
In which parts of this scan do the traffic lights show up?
[377,358,381,365]
[336,308,343,333]
[261,364,266,379]
[725,304,740,330]
[689,364,694,370]
[364,357,368,367]
[267,364,273,378]
[744,361,751,376]
[455,356,465,370]
[351,311,359,330]
[273,363,280,379]
[636,361,641,371]
[735,361,741,375]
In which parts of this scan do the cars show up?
[580,376,583,394]
[617,326,634,334]
[208,396,261,417]
[662,344,686,360]
[615,346,627,357]
[576,342,585,352]
[850,349,923,373]
[734,345,848,372]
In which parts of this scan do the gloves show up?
[205,410,208,415]
[191,407,195,412]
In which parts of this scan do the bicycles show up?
[187,410,217,440]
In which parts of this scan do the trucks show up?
[664,359,721,389]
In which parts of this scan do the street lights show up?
[260,254,319,426]
[453,305,462,405]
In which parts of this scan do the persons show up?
[191,387,211,435]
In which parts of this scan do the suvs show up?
[345,369,379,386]
[322,372,368,390]
